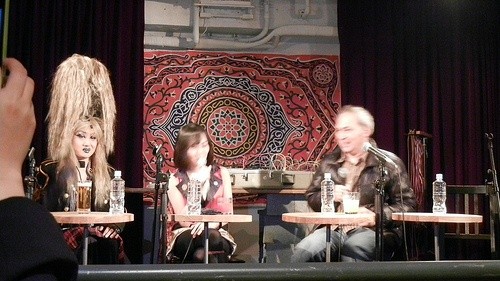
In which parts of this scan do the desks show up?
[49,188,483,265]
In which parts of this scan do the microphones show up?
[362,142,399,168]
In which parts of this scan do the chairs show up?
[444,185,495,259]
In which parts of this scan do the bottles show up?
[110,171,125,214]
[187,176,201,215]
[433,174,447,214]
[321,173,335,213]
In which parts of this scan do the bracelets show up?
[216,221,224,230]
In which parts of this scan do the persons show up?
[163,122,236,263]
[291,105,415,262]
[33,115,132,264]
[0,57,79,280]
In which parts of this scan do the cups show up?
[342,192,360,213]
[77,180,91,214]
